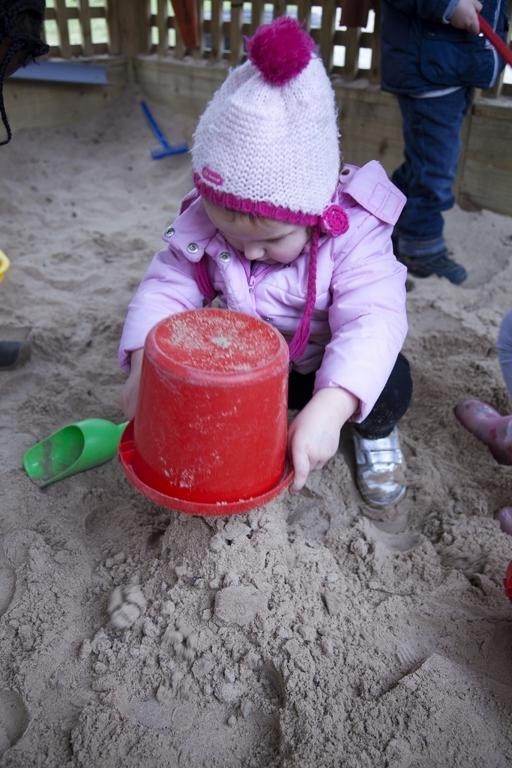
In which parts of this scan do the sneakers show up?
[494,506,512,534]
[349,426,407,507]
[454,398,512,464]
[394,249,467,283]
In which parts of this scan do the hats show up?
[190,14,348,237]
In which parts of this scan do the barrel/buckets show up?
[120,309,295,518]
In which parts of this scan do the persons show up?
[0,0,52,370]
[370,0,512,285]
[117,13,414,509]
[451,311,511,533]
